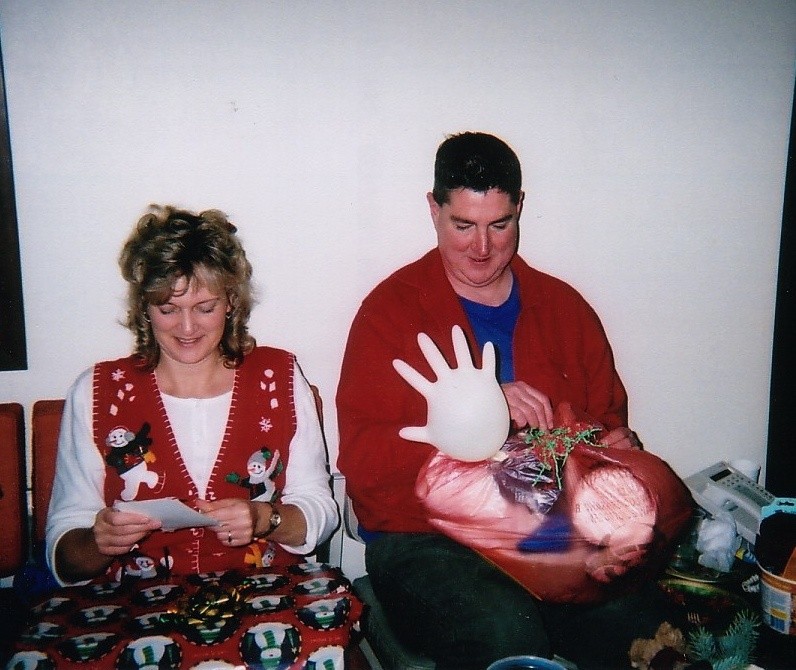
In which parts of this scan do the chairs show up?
[25,384,331,575]
[332,465,443,670]
[0,400,31,583]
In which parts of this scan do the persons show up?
[0,205,364,670]
[336,131,666,669]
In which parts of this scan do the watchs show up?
[255,502,282,538]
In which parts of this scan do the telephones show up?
[682,460,775,544]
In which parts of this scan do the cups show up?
[730,460,761,484]
[486,655,566,670]
[754,561,796,637]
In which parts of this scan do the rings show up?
[228,531,232,542]
